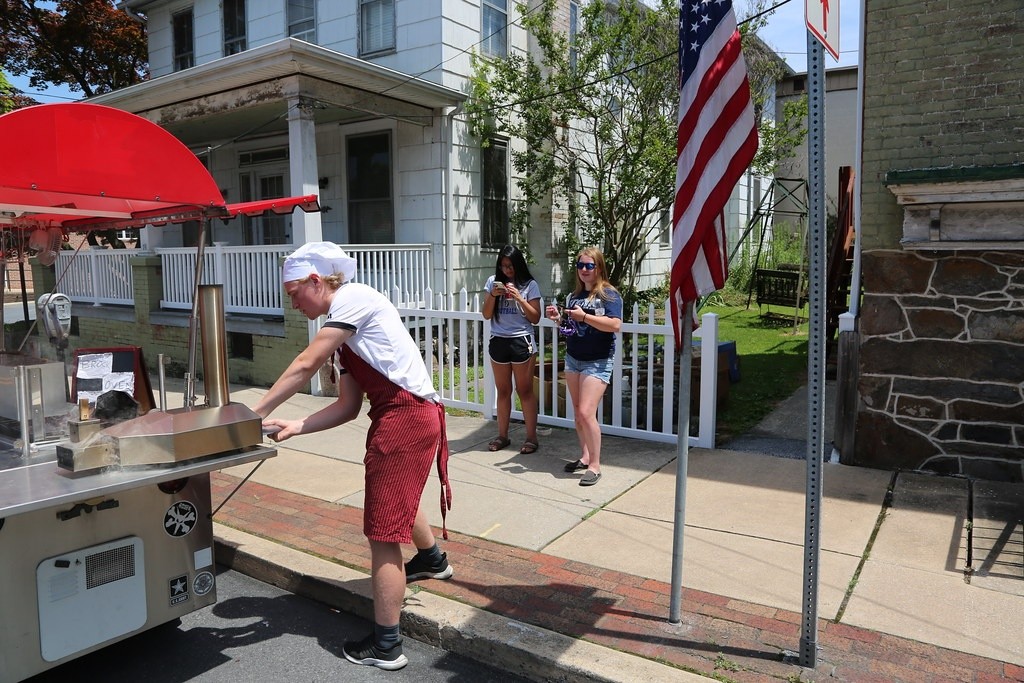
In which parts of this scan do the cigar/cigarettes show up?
[331,609,340,613]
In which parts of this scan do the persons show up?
[250,241,452,670]
[546,247,622,485]
[482,244,541,454]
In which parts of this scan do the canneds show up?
[505,283,514,300]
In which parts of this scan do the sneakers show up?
[404,551,453,580]
[579,470,602,485]
[563,459,589,473]
[342,630,408,671]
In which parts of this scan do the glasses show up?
[499,263,513,270]
[576,261,597,270]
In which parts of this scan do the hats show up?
[281,241,357,284]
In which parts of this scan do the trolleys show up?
[0,103,329,683]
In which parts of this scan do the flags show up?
[669,0,759,355]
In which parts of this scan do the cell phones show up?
[493,281,508,293]
[564,307,574,310]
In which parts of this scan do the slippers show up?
[489,436,511,451]
[520,440,538,454]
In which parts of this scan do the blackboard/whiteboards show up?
[71,346,156,412]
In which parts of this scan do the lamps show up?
[319,177,328,188]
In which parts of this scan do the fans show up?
[29,220,64,267]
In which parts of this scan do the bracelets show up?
[583,313,586,322]
[490,292,495,297]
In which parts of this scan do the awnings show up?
[0,103,330,411]
